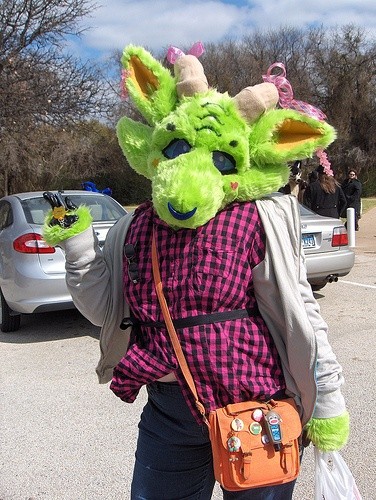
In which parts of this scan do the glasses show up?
[124,241,139,284]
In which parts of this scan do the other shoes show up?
[356,228,359,231]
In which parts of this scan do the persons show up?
[42,41,350,500]
[277,160,362,232]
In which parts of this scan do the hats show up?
[316,165,337,175]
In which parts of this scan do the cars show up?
[0,189,129,333]
[275,192,355,291]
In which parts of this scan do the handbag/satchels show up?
[314,448,363,500]
[208,398,302,491]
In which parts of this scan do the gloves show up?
[302,430,316,447]
[43,192,79,228]
